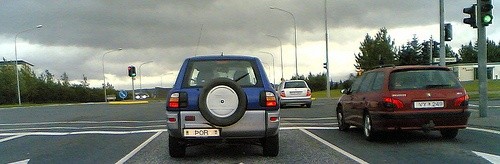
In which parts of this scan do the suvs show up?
[165,55,280,160]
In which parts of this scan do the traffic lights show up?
[481,1,493,25]
[464,6,476,28]
[445,23,452,40]
[128,66,136,77]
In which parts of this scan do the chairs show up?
[195,69,214,85]
[234,70,250,84]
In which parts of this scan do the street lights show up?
[266,34,284,83]
[14,23,42,107]
[102,48,124,103]
[161,72,173,99]
[140,60,153,100]
[269,7,298,78]
[258,50,276,88]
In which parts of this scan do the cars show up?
[277,81,313,108]
[336,63,470,138]
